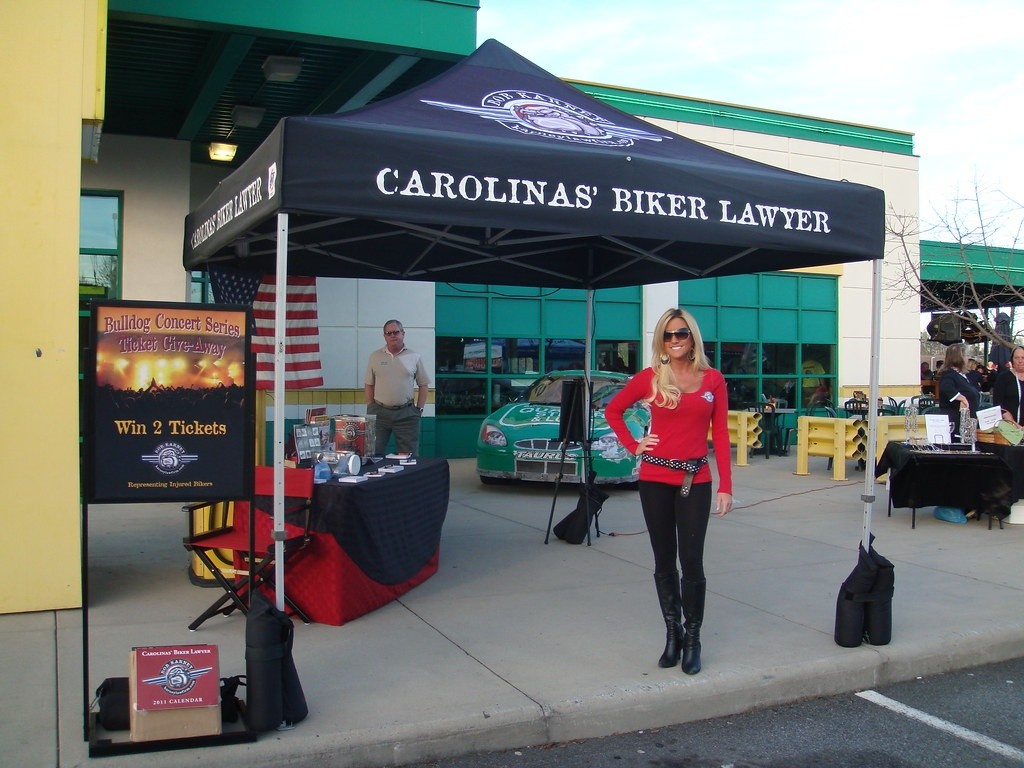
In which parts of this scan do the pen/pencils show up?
[385,464,393,468]
[395,453,410,455]
[407,457,412,462]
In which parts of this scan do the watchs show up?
[417,408,423,412]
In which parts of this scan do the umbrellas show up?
[989,312,1012,367]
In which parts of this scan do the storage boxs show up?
[128,640,223,740]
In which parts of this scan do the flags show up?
[207,266,323,390]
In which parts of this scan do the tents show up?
[183,39,895,728]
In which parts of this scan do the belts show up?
[375,399,413,409]
[642,452,710,498]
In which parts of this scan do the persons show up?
[966,359,1011,394]
[935,344,979,429]
[605,309,732,675]
[934,360,943,376]
[993,346,1024,429]
[803,352,828,407]
[922,362,933,394]
[365,320,429,455]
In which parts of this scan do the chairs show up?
[742,395,934,475]
[184,467,320,634]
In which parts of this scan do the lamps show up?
[208,141,241,162]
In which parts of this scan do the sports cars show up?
[475,368,649,492]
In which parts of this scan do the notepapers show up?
[338,476,369,483]
[363,472,385,477]
[400,459,416,465]
[378,465,404,473]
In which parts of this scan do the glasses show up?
[384,330,400,336]
[663,328,691,342]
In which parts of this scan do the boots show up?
[653,571,684,668]
[680,576,706,675]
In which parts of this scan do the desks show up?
[875,441,1014,530]
[244,457,454,626]
[976,441,1024,502]
